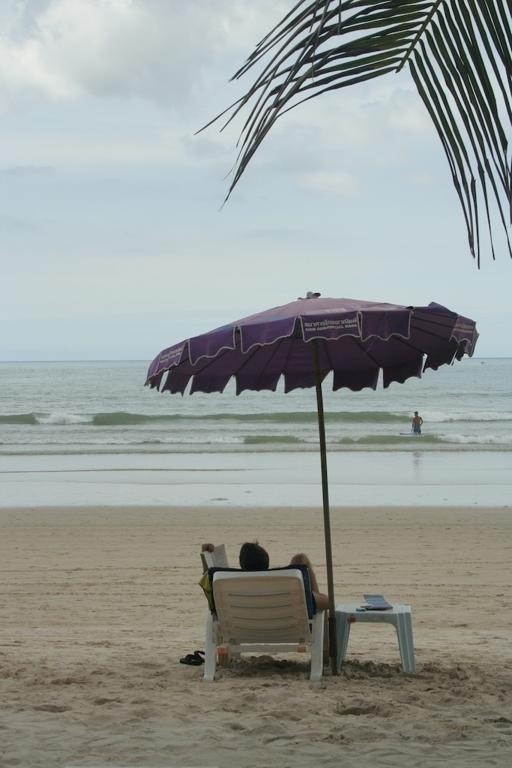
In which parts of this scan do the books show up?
[360,594,393,610]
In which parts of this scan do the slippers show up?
[180,650,205,666]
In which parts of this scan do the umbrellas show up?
[144,292,479,676]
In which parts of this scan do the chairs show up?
[202,543,326,675]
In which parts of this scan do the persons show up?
[200,543,329,633]
[412,411,423,433]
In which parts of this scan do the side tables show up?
[330,599,415,677]
